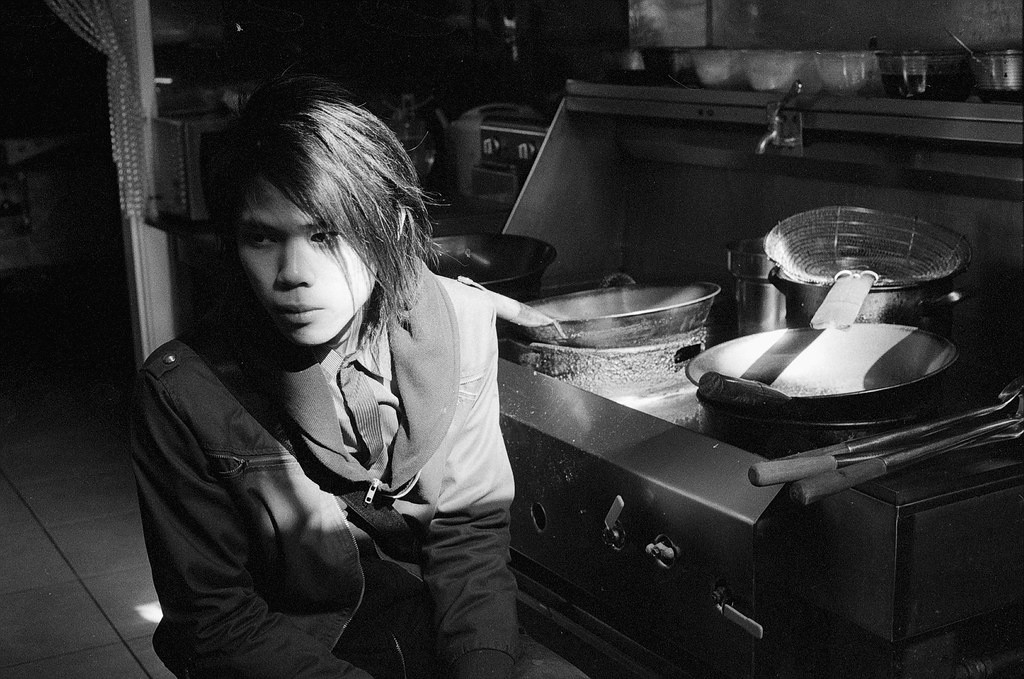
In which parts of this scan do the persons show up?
[128,77,591,679]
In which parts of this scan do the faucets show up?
[756,80,803,155]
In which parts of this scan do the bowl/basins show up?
[973,53,1024,105]
[689,50,740,86]
[741,48,798,93]
[793,50,821,93]
[817,51,876,96]
[876,52,966,96]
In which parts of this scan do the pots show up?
[421,234,556,292]
[458,271,720,344]
[688,324,960,416]
[769,245,977,329]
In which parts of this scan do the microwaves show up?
[151,114,249,223]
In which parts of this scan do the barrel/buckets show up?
[725,239,770,338]
